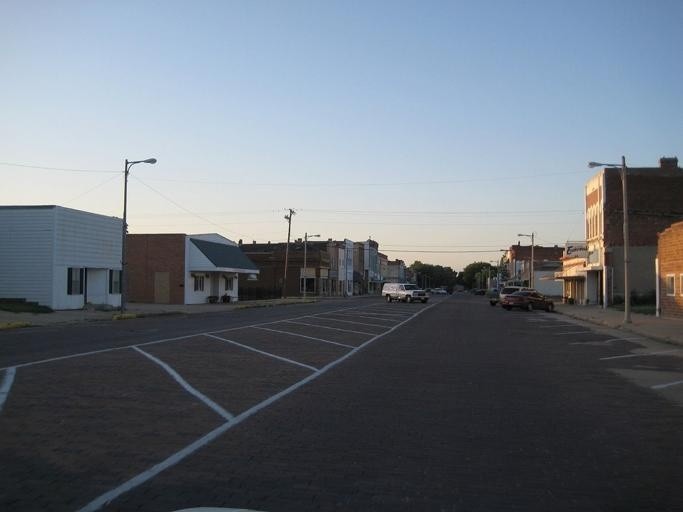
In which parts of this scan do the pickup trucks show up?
[382,282,429,305]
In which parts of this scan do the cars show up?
[430,288,446,296]
[501,289,556,312]
[470,288,484,295]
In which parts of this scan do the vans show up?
[498,285,534,304]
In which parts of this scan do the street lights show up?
[517,232,536,290]
[490,259,499,288]
[119,158,160,317]
[586,152,631,324]
[302,231,321,300]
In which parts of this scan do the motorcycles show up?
[487,289,499,307]
